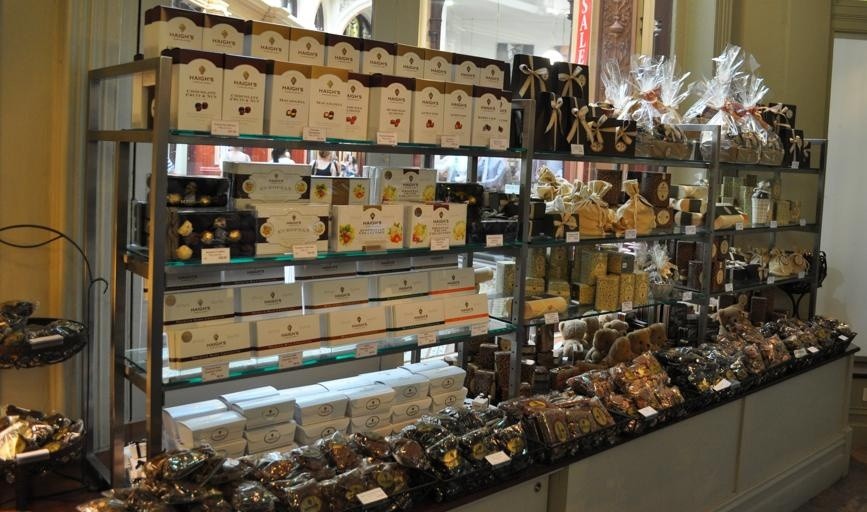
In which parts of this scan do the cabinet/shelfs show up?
[0,217,108,512]
[558,339,856,512]
[431,460,558,512]
[80,55,830,492]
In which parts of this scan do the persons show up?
[269,148,295,165]
[309,150,359,176]
[221,146,250,176]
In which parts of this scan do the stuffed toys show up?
[557,312,668,366]
[718,306,752,336]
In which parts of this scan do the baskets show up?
[344,436,546,510]
[550,332,859,467]
